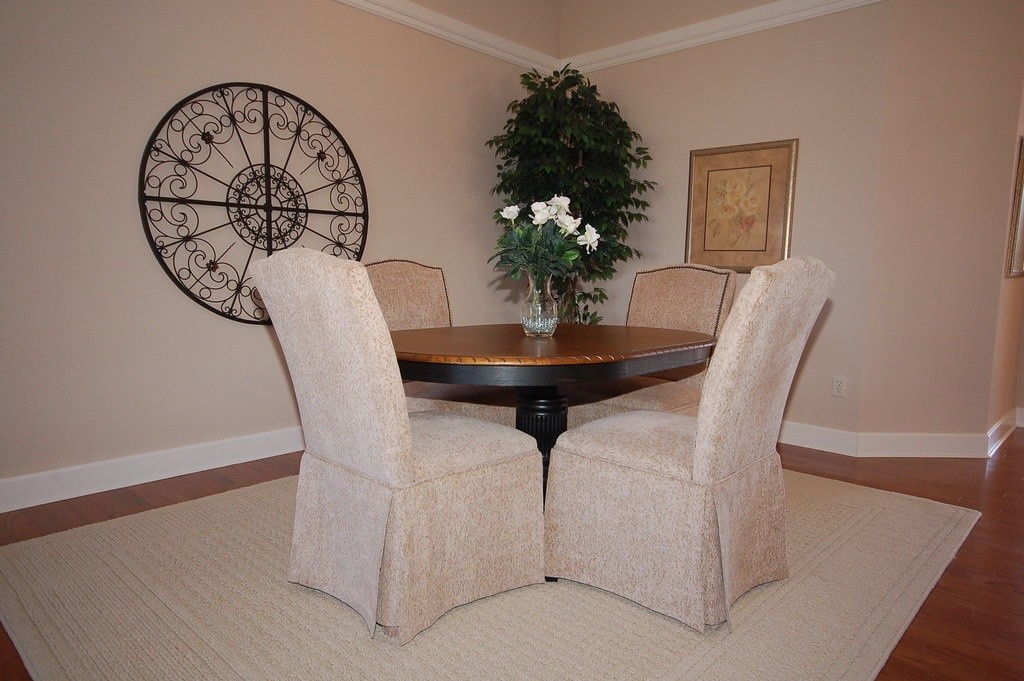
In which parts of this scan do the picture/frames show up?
[685,138,798,272]
[1005,135,1024,276]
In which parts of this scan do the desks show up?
[387,323,718,584]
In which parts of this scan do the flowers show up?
[488,193,600,311]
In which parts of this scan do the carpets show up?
[1,467,983,681]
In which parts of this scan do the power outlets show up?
[832,376,847,398]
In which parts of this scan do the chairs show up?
[567,265,736,433]
[546,255,835,635]
[364,259,516,431]
[247,247,547,645]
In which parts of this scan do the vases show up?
[520,274,558,338]
